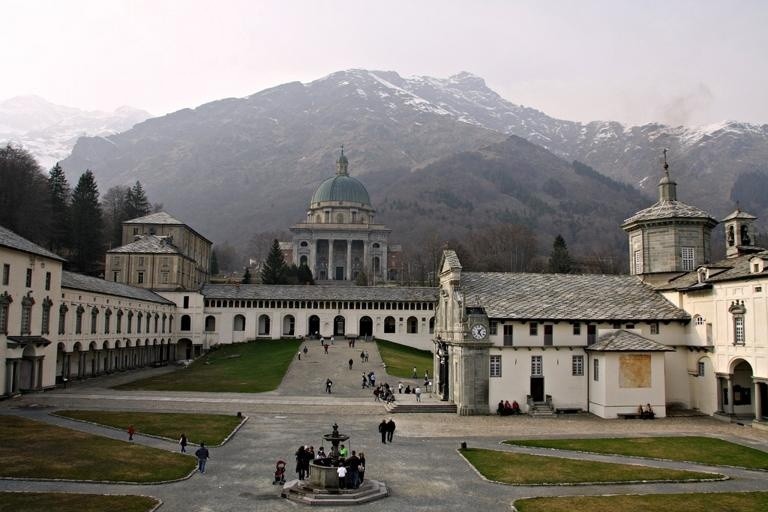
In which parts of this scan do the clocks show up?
[469,322,488,341]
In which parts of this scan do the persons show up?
[638,405,643,417]
[646,404,654,417]
[297,330,422,402]
[512,400,519,414]
[292,444,366,489]
[179,433,187,452]
[127,423,135,439]
[194,441,210,472]
[498,400,504,415]
[505,400,513,416]
[378,419,386,443]
[387,419,395,442]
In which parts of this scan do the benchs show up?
[616,412,656,419]
[496,409,522,416]
[556,408,583,416]
